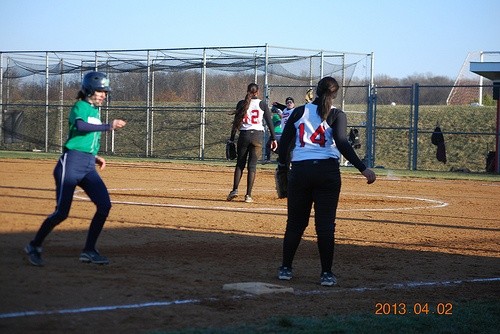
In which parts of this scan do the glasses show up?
[286,101,293,105]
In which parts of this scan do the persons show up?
[24,72,126,266]
[269,98,295,134]
[275,77,376,285]
[227,83,278,202]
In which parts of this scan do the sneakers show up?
[227,189,239,200]
[244,195,254,202]
[320,272,336,285]
[24,240,46,266]
[80,248,108,264]
[277,265,292,280]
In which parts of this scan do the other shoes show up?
[265,159,270,163]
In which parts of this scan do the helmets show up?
[82,72,112,97]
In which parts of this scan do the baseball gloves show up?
[226,142,238,161]
[276,168,292,199]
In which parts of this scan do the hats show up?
[286,97,294,103]
